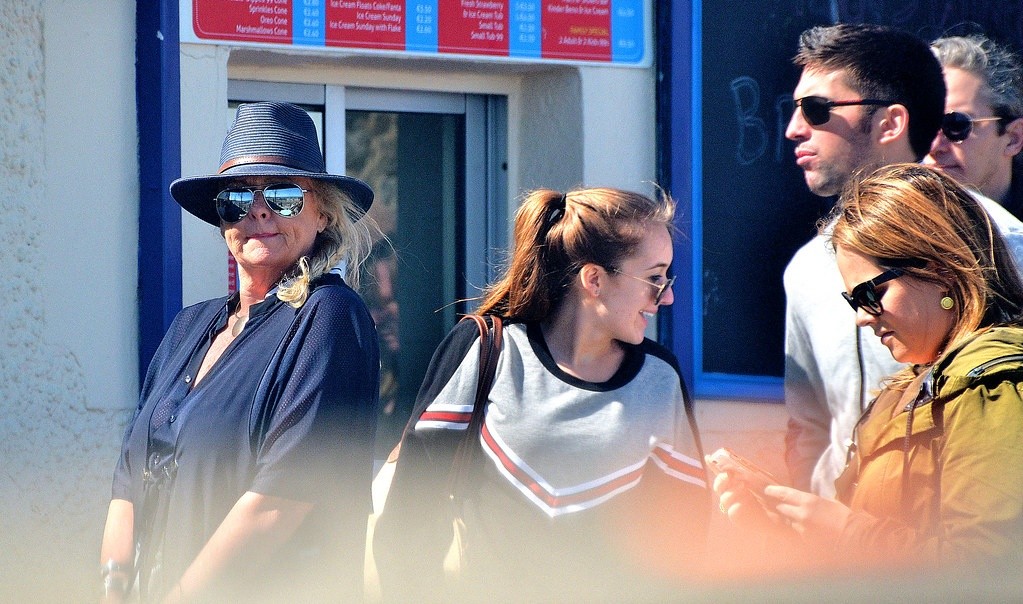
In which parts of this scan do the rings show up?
[719,505,728,513]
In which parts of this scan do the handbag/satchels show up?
[360,313,502,604]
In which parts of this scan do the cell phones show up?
[710,447,778,492]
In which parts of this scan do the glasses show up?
[213,180,316,225]
[938,111,1002,143]
[841,268,909,315]
[609,270,677,305]
[780,96,902,127]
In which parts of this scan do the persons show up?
[373,187,710,604]
[101,100,381,604]
[360,232,400,418]
[714,22,1023,604]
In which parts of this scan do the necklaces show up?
[232,301,249,337]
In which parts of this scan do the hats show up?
[169,102,374,228]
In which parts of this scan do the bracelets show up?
[102,560,132,595]
